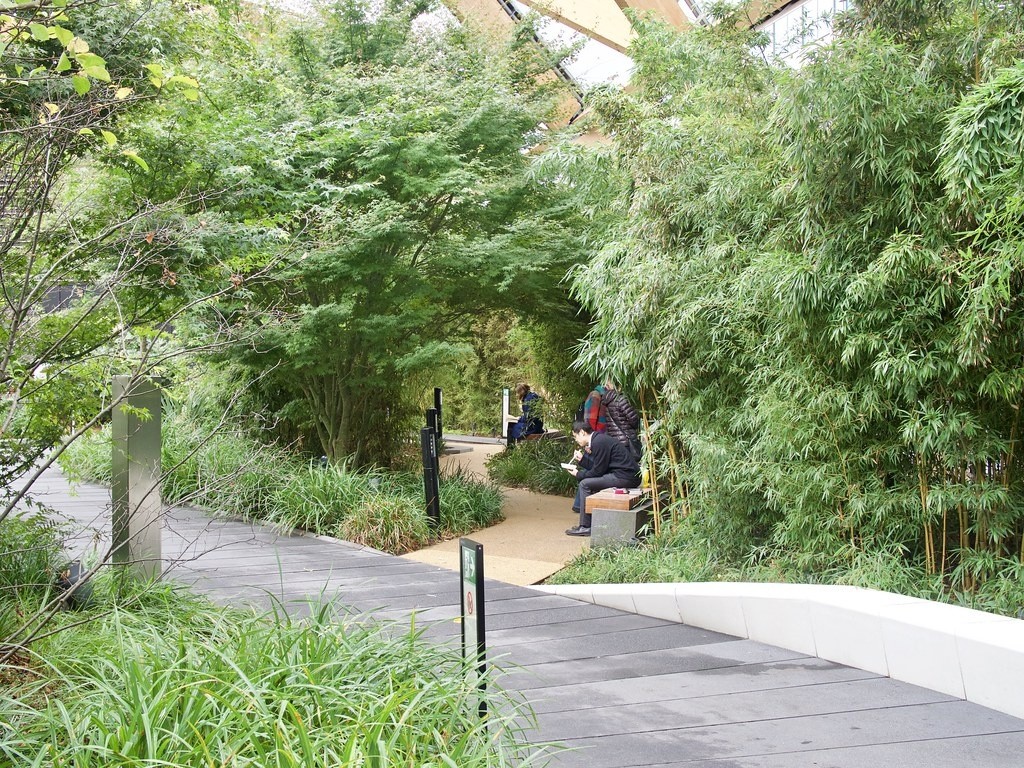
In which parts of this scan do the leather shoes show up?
[566,525,591,536]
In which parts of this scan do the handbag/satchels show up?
[606,405,643,462]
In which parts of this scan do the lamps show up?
[426,408,439,433]
[421,427,438,468]
[434,387,442,411]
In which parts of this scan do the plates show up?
[561,463,577,470]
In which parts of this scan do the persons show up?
[572,373,640,513]
[507,384,545,451]
[565,421,642,537]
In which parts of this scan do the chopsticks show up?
[568,449,581,464]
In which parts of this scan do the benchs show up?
[521,428,563,445]
[585,485,670,554]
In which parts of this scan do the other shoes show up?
[572,507,580,512]
